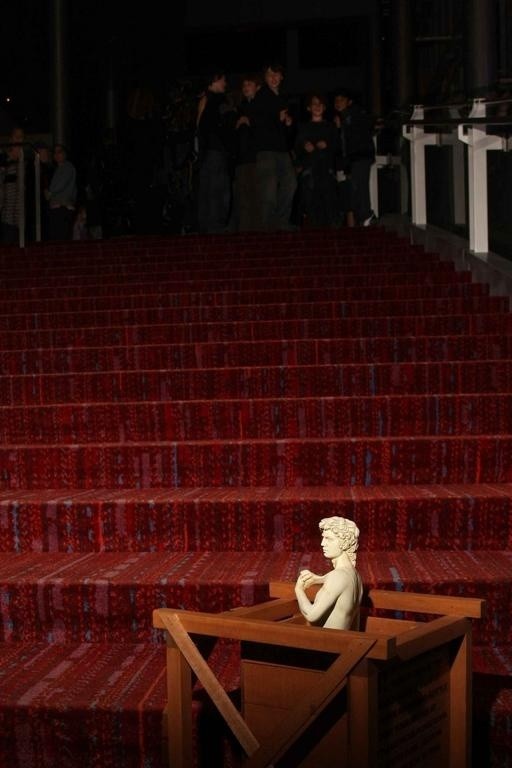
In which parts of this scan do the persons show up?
[294,515,364,632]
[0,126,91,244]
[195,61,378,233]
